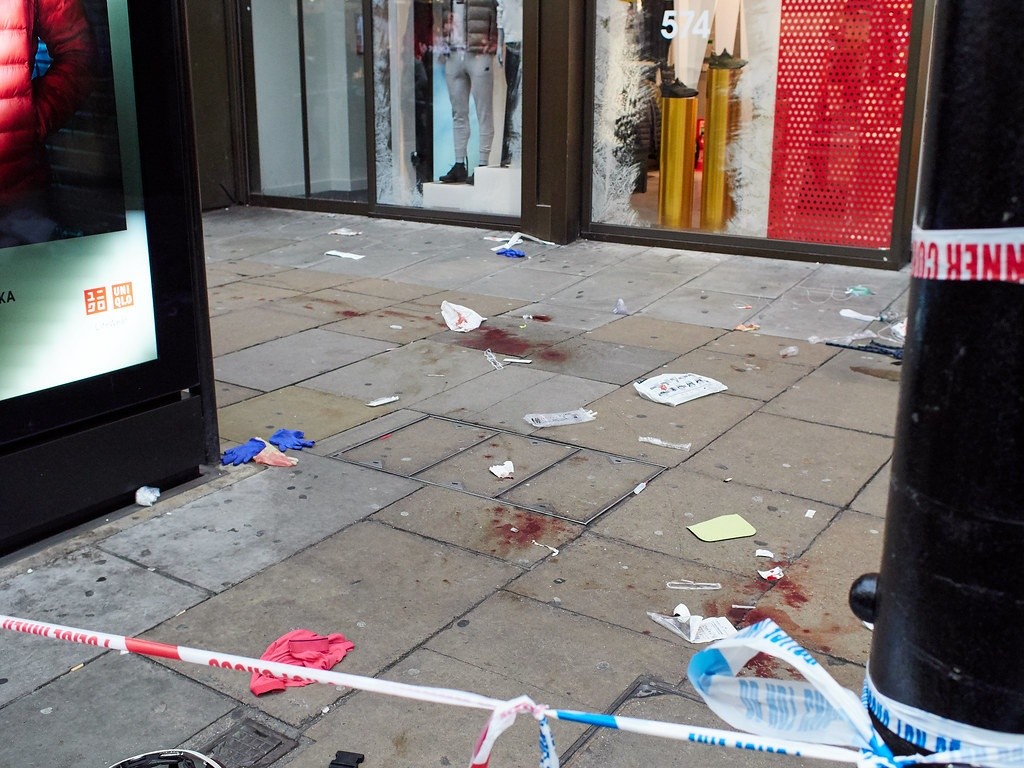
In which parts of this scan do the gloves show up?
[220,438,266,466]
[496,249,525,258]
[269,429,315,452]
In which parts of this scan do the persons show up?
[0,0,102,249]
[436,0,523,184]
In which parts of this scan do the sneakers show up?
[466,173,474,184]
[439,162,467,182]
[709,48,745,69]
[661,77,698,98]
